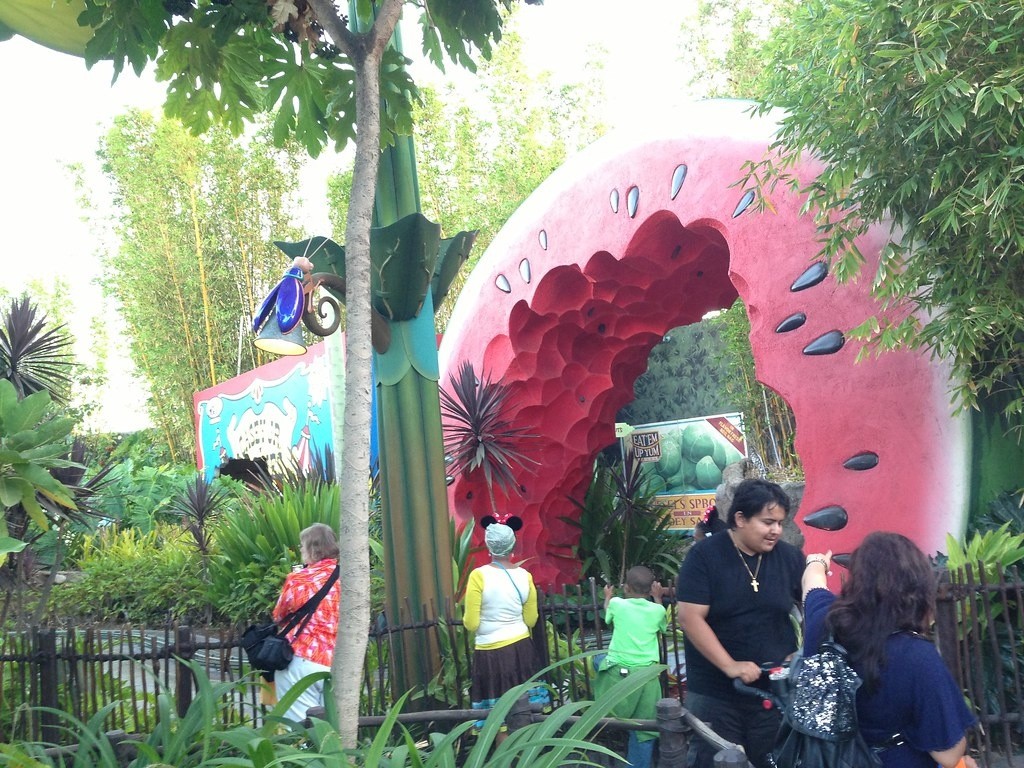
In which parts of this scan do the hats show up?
[485,523,516,556]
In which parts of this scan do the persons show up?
[273,522,339,742]
[462,524,539,751]
[675,477,806,768]
[604,568,666,766]
[802,533,977,768]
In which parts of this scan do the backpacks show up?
[766,624,905,768]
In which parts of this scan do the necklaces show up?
[730,530,762,592]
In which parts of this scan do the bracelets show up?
[805,556,828,574]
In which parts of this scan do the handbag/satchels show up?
[257,636,293,670]
[241,624,280,682]
